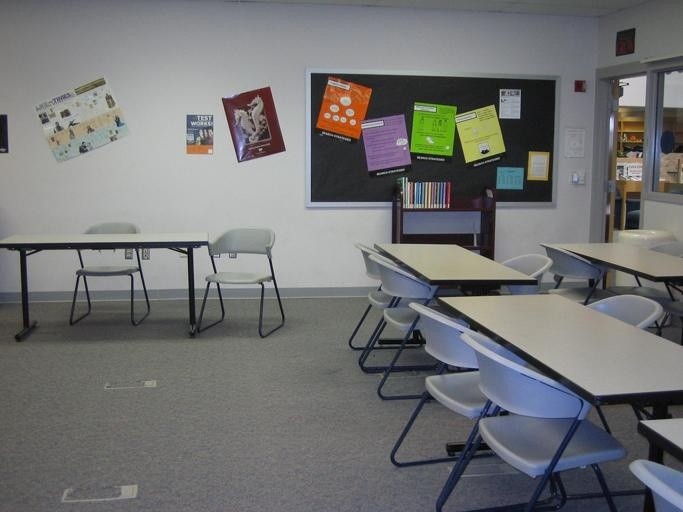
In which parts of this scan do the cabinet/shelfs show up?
[392,188,497,261]
[619,106,683,156]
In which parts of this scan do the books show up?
[416,183,419,208]
[447,182,452,209]
[405,176,408,208]
[425,181,428,208]
[422,182,425,208]
[440,182,443,208]
[434,181,437,208]
[437,182,440,208]
[410,181,414,209]
[396,177,405,208]
[418,182,422,208]
[443,182,447,209]
[408,181,410,208]
[413,183,416,209]
[431,182,435,208]
[428,182,431,208]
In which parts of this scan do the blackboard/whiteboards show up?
[305,68,561,208]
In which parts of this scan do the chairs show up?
[586,294,663,435]
[391,302,558,512]
[436,332,628,512]
[501,253,553,295]
[69,221,150,326]
[196,227,285,338]
[349,240,439,370]
[629,458,683,512]
[360,255,437,401]
[539,242,617,305]
[607,241,683,328]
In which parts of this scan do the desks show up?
[0,232,208,342]
[638,419,683,510]
[439,293,683,461]
[615,181,669,230]
[551,244,683,287]
[374,244,539,344]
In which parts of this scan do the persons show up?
[54,121,65,133]
[106,93,115,108]
[195,128,204,143]
[201,126,213,145]
[115,115,124,127]
[200,127,207,144]
[79,142,89,154]
[68,119,80,128]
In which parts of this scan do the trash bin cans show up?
[617,226,674,327]
